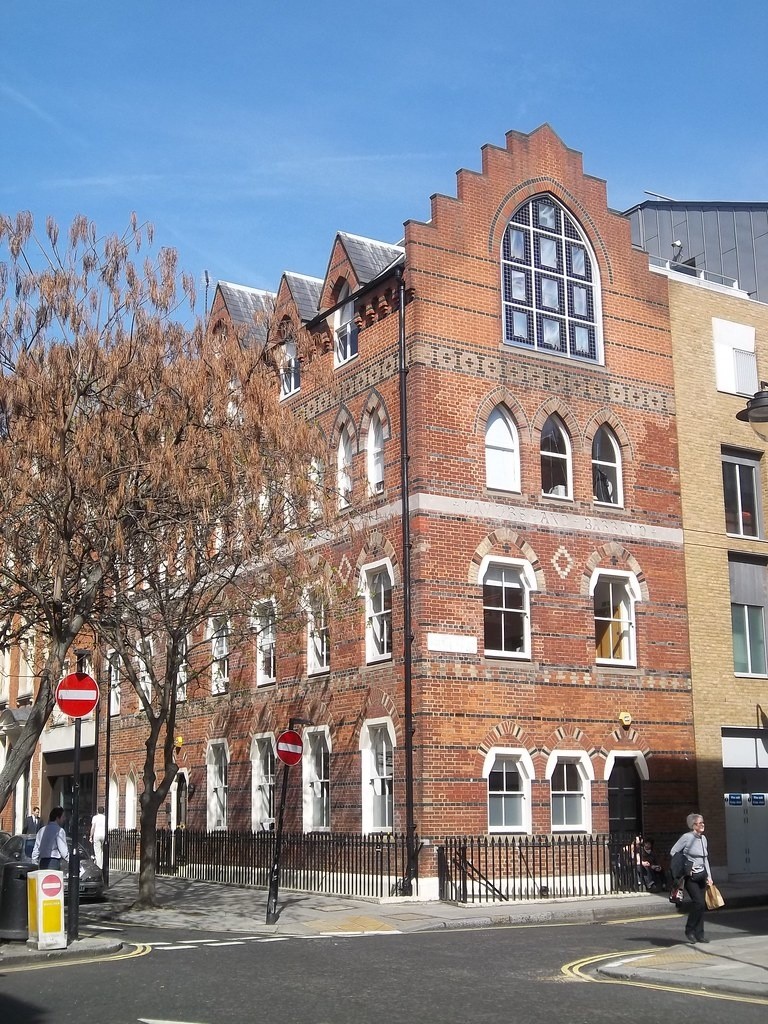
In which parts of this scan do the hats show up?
[646,838,655,844]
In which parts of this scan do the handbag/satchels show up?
[705,884,726,909]
[692,869,708,882]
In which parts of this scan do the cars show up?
[0,833,105,900]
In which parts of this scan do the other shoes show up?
[697,935,709,943]
[684,931,696,944]
[648,884,662,893]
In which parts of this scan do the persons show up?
[89,806,105,871]
[31,806,69,871]
[639,838,667,889]
[23,807,44,834]
[669,814,713,944]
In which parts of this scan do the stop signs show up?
[38,874,63,899]
[54,672,100,718]
[276,729,303,766]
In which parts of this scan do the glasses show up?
[694,821,704,825]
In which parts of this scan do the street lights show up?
[735,381,768,443]
